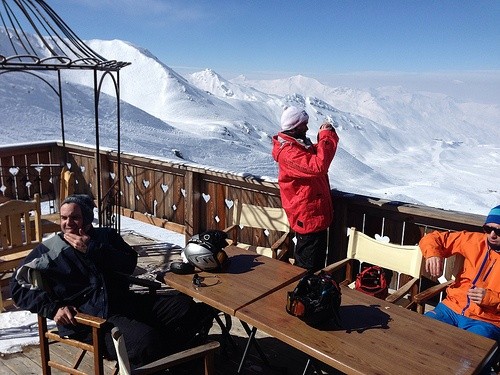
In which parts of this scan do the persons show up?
[272,106,339,272]
[419,205,500,343]
[9,194,211,375]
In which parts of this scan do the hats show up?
[280,106,309,130]
[61,194,95,229]
[485,204,500,224]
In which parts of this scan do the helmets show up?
[354,264,388,301]
[294,270,341,316]
[184,230,229,270]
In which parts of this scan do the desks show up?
[155,246,306,375]
[235,275,500,375]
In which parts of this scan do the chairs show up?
[0,167,454,375]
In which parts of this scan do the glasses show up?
[286,293,304,316]
[483,224,500,237]
[214,250,228,267]
